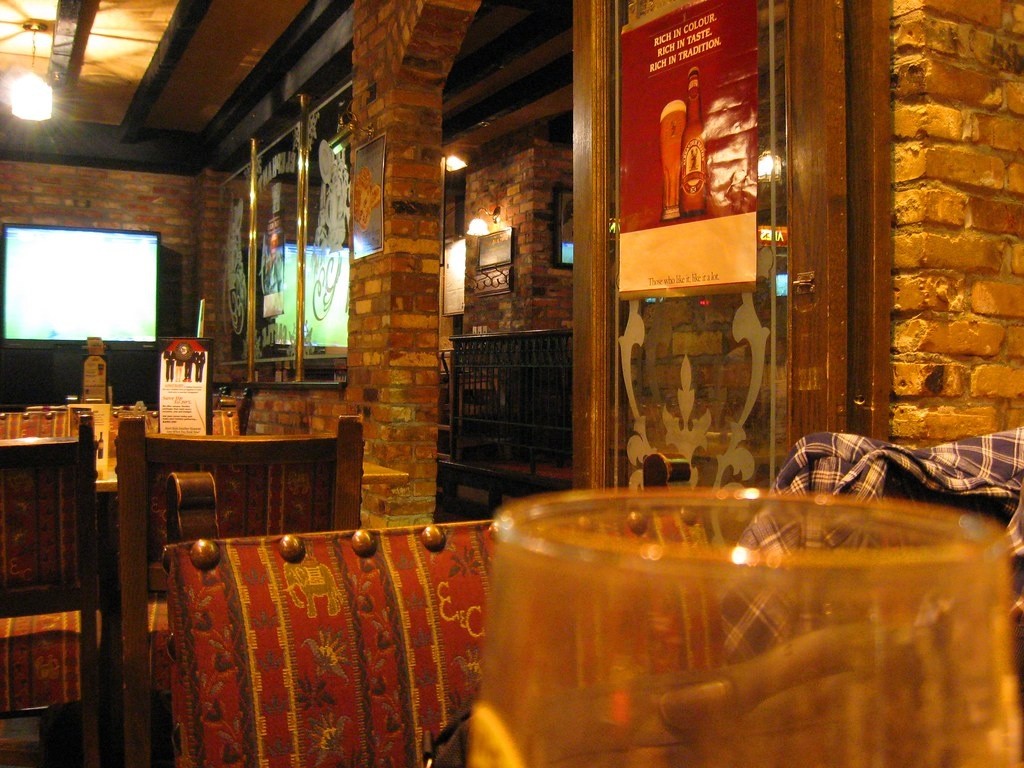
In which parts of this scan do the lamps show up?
[464,205,504,237]
[758,150,783,184]
[9,23,53,122]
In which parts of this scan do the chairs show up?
[0,406,103,767]
[0,409,94,439]
[47,414,366,768]
[109,397,242,462]
[0,396,80,440]
[215,386,251,436]
[160,516,494,768]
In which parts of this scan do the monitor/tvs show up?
[0,223,160,350]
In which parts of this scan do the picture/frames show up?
[475,226,515,272]
[439,236,466,318]
[552,183,573,271]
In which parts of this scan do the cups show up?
[465,485,1024,768]
[657,98,688,223]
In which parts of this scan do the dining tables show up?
[96,457,410,492]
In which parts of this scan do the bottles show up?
[259,181,286,295]
[678,64,709,217]
